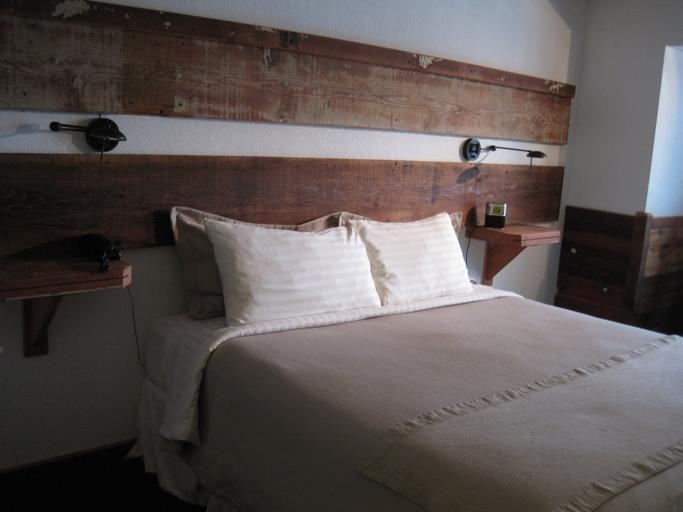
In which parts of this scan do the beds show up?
[119,202,683,511]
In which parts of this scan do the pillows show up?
[204,217,387,327]
[170,206,341,320]
[349,211,474,315]
[336,212,464,238]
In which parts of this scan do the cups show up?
[485,202,506,229]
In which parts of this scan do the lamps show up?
[50,113,127,153]
[462,138,546,161]
